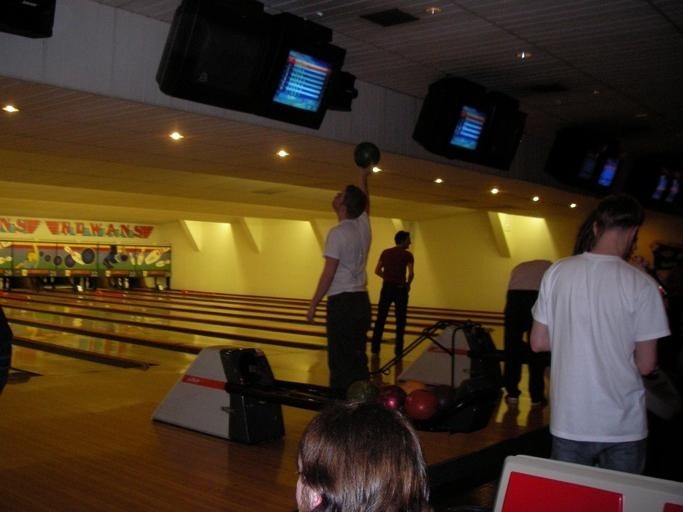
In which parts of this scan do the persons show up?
[528,195,673,473]
[372,230,415,356]
[572,211,650,273]
[500,259,554,408]
[293,395,431,512]
[306,162,372,400]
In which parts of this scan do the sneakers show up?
[504,393,519,401]
[530,399,545,407]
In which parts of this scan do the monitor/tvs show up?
[582,138,621,196]
[156,0,274,115]
[472,89,528,171]
[413,73,489,163]
[657,163,683,212]
[254,13,346,129]
[624,158,671,209]
[0,0,57,39]
[544,127,603,188]
